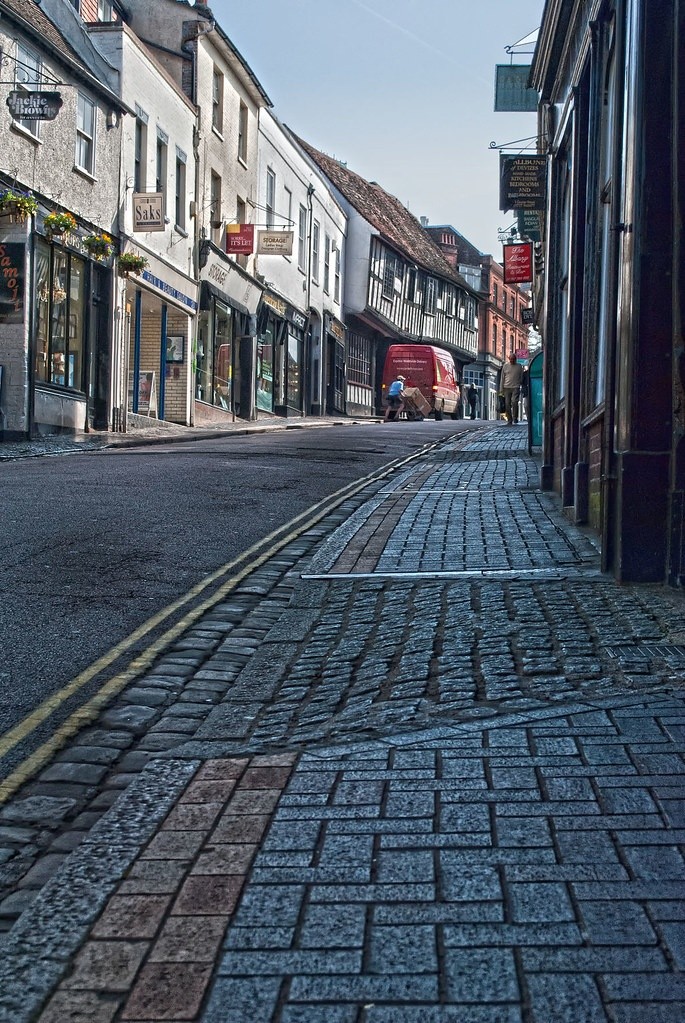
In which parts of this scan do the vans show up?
[217,344,231,387]
[381,344,463,421]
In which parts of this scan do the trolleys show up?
[398,389,423,421]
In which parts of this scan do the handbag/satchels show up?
[495,396,506,413]
[472,393,479,403]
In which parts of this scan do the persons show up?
[500,354,524,425]
[166,338,177,360]
[141,373,152,402]
[384,374,406,422]
[468,383,478,420]
[521,359,533,420]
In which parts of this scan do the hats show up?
[397,375,406,379]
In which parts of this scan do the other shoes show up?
[507,421,512,425]
[514,418,518,423]
[471,417,475,420]
[384,418,391,421]
[392,418,398,421]
